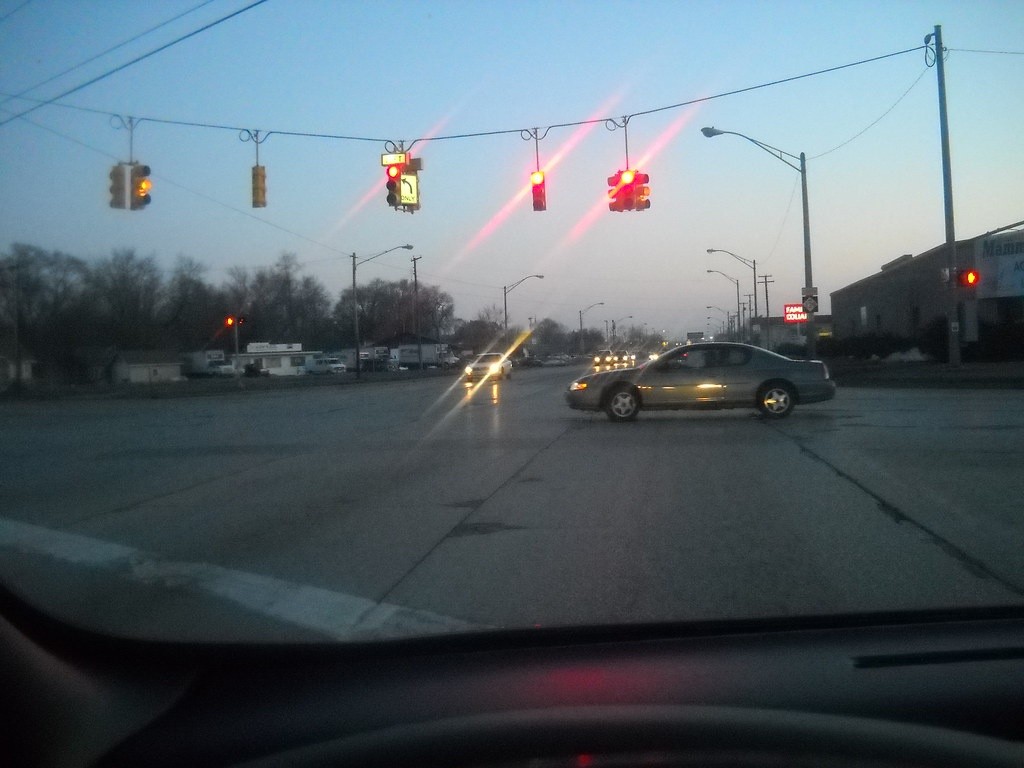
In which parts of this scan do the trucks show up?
[178,349,234,377]
[398,343,459,371]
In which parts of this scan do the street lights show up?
[698,127,817,361]
[579,302,605,353]
[351,244,415,380]
[504,274,544,351]
[706,249,762,346]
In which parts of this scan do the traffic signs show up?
[399,173,417,204]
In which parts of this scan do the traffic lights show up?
[962,271,980,286]
[108,165,125,209]
[385,163,402,207]
[607,170,624,211]
[632,171,651,211]
[530,171,547,211]
[133,162,151,211]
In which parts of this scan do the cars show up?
[306,358,345,374]
[505,343,667,367]
[465,352,513,382]
[564,342,836,422]
